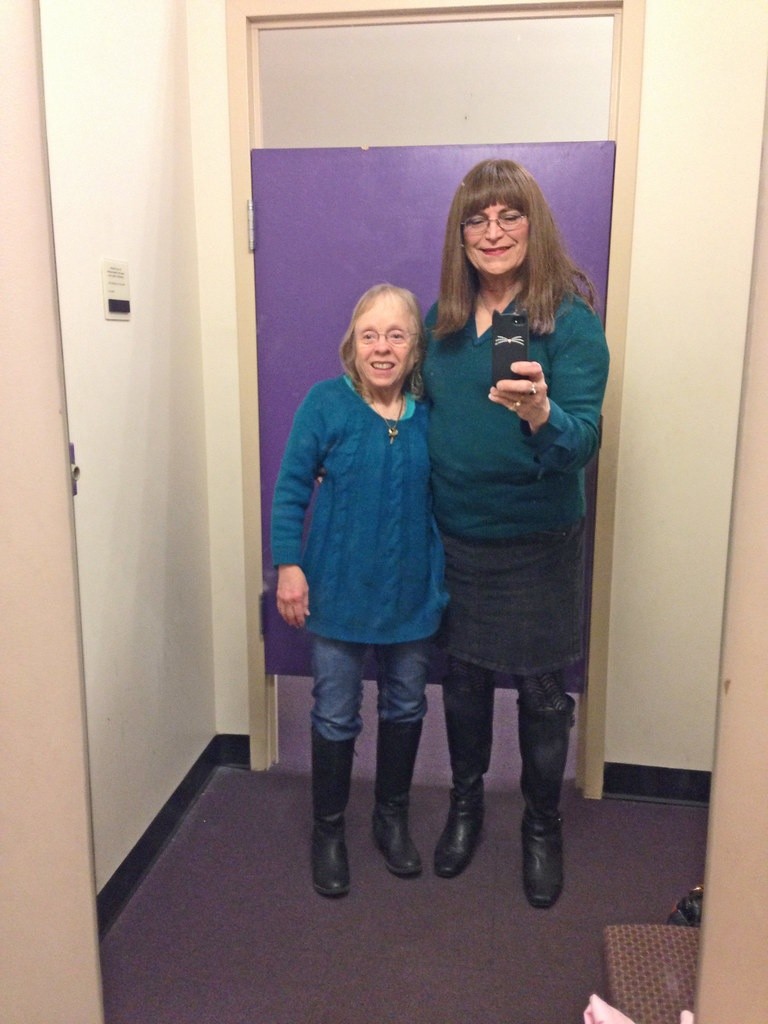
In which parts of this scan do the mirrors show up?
[37,0,765,1024]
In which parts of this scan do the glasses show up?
[461,211,528,234]
[354,330,417,347]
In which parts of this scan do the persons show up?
[271,283,450,896]
[318,157,610,909]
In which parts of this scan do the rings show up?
[514,400,521,406]
[529,384,537,395]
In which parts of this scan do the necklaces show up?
[372,394,404,444]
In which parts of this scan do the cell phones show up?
[492,308,529,388]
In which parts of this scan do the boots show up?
[435,680,495,878]
[516,693,576,908]
[371,717,424,874]
[311,725,355,895]
